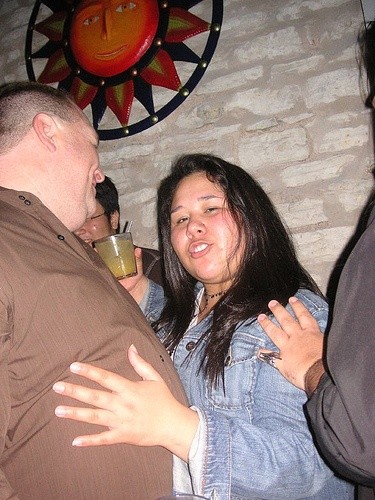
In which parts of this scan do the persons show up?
[72,177,166,297]
[54,155,359,500]
[260,18,374,500]
[0,85,194,499]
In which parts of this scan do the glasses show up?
[84,211,108,226]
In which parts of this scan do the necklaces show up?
[199,291,228,315]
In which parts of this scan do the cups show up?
[94,231,138,281]
